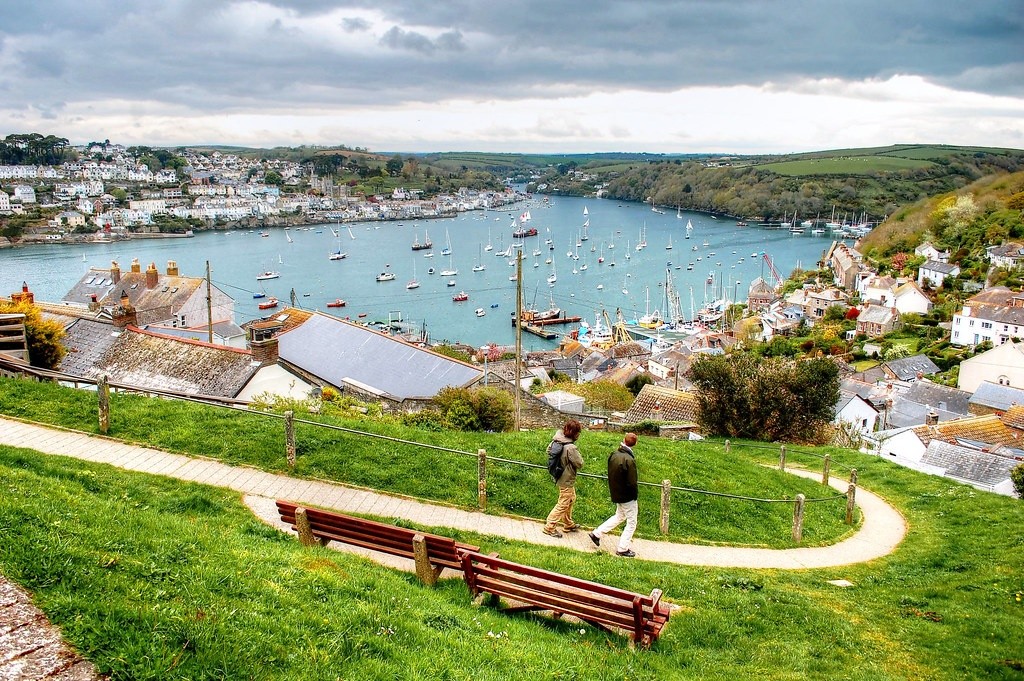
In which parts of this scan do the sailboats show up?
[568,271,732,354]
[407,257,420,290]
[411,227,486,276]
[781,203,872,238]
[685,220,693,239]
[666,234,673,250]
[484,208,647,285]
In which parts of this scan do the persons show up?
[542,420,584,537]
[589,432,638,557]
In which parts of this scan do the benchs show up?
[458,551,676,650]
[275,498,480,586]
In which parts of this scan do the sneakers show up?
[542,527,562,538]
[563,524,580,532]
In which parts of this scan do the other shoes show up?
[616,549,636,557]
[589,532,600,547]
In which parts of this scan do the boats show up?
[651,198,665,214]
[256,259,280,280]
[511,274,582,339]
[448,279,455,286]
[224,230,270,238]
[284,213,356,261]
[376,272,395,281]
[452,291,469,302]
[252,291,279,309]
[327,298,346,307]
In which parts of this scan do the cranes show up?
[760,251,788,286]
[666,269,682,327]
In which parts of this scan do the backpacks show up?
[547,439,574,487]
